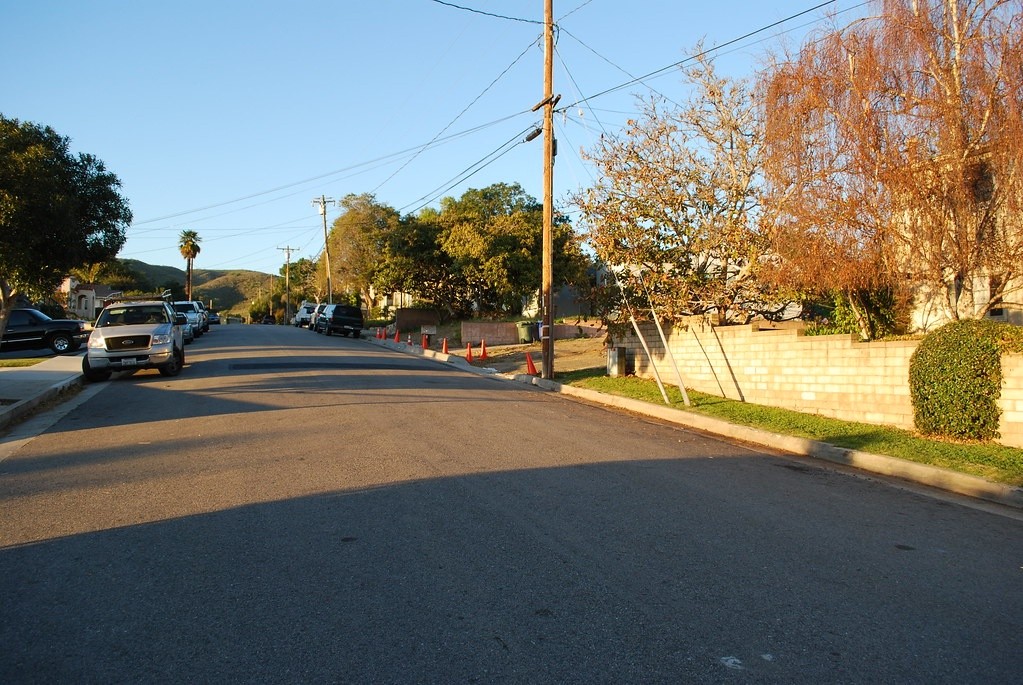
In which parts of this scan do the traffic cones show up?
[477,339,489,359]
[394,330,399,343]
[382,328,387,340]
[526,352,538,376]
[442,338,449,354]
[407,333,412,346]
[376,328,381,339]
[466,343,473,363]
[423,334,427,349]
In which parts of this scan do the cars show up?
[263,315,276,325]
[170,301,204,338]
[209,314,220,324]
[308,303,327,332]
[196,301,210,332]
[0,308,95,354]
[295,305,317,328]
[82,301,188,383]
[174,312,194,345]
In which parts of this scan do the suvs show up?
[317,304,364,339]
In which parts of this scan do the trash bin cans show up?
[515,320,543,344]
